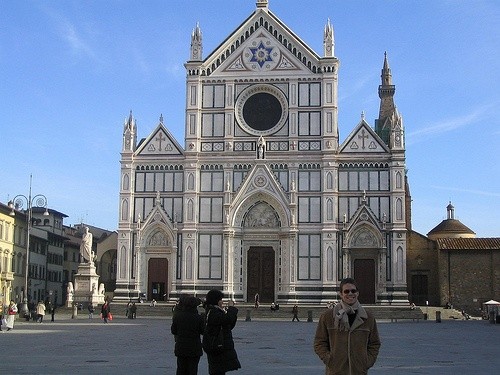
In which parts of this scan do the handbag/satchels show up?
[108,312,112,320]
[203,323,225,353]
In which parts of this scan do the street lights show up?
[10,174,50,318]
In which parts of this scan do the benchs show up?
[388,318,421,322]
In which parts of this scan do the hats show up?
[206,289,224,303]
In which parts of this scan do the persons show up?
[292,305,300,322]
[171,294,206,375]
[462,309,470,320]
[410,303,416,311]
[0,296,158,332]
[446,302,451,310]
[80,226,92,263]
[255,293,279,312]
[314,277,381,375]
[203,289,241,375]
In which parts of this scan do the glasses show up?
[344,288,357,293]
